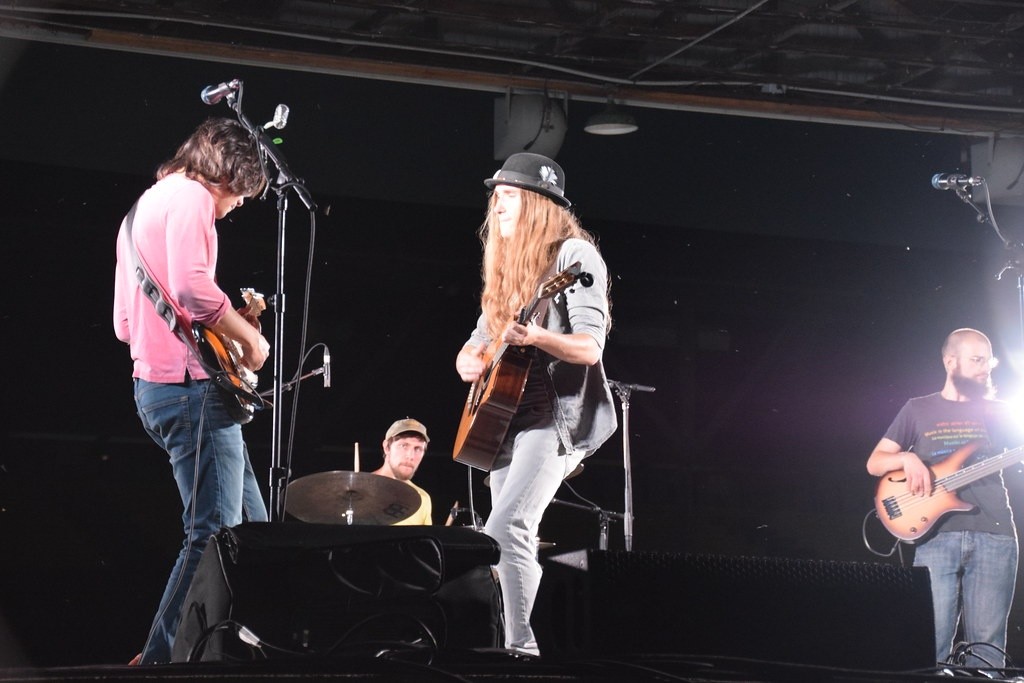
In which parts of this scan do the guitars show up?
[451,262,594,473]
[190,285,267,426]
[874,435,1024,544]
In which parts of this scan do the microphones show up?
[201,78,242,105]
[322,346,331,388]
[606,379,656,393]
[931,173,984,191]
[273,103,290,129]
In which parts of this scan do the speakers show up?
[528,551,937,671]
[171,522,506,662]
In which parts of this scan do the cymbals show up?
[278,470,422,525]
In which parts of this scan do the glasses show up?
[947,352,999,368]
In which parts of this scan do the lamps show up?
[967,131,1023,208]
[582,89,639,136]
[493,78,572,163]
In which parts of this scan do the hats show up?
[480,153,574,209]
[384,416,430,443]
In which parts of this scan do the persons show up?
[865,329,1024,678]
[457,152,617,661]
[364,419,432,526]
[111,121,276,665]
